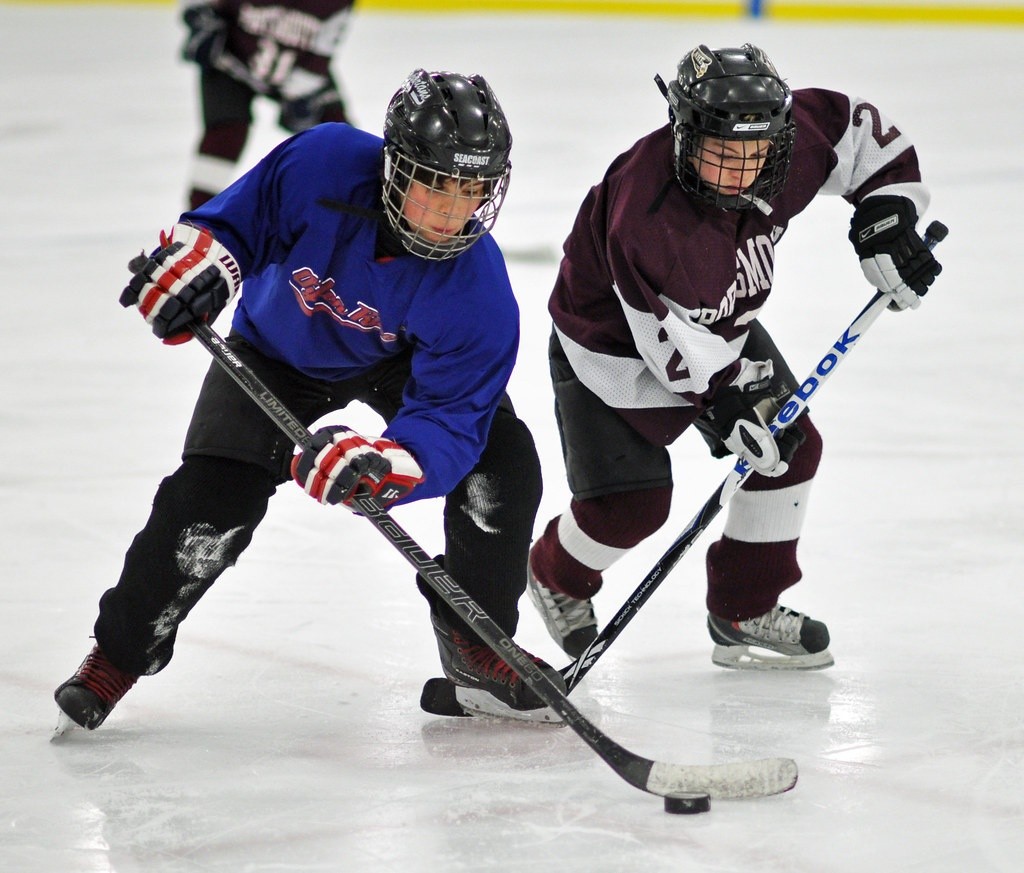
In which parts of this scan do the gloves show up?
[120,223,242,345]
[706,360,808,479]
[848,195,942,310]
[279,86,329,135]
[187,4,242,68]
[289,428,423,516]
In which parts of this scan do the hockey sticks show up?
[126,252,799,803]
[419,219,950,718]
[213,55,272,94]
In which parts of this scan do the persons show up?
[181,0,361,208]
[525,44,943,673]
[52,72,572,744]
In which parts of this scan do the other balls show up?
[664,791,712,816]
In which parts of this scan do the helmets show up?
[381,69,514,260]
[653,43,794,212]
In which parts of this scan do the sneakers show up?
[707,599,834,672]
[531,556,597,658]
[417,557,567,724]
[52,640,134,737]
